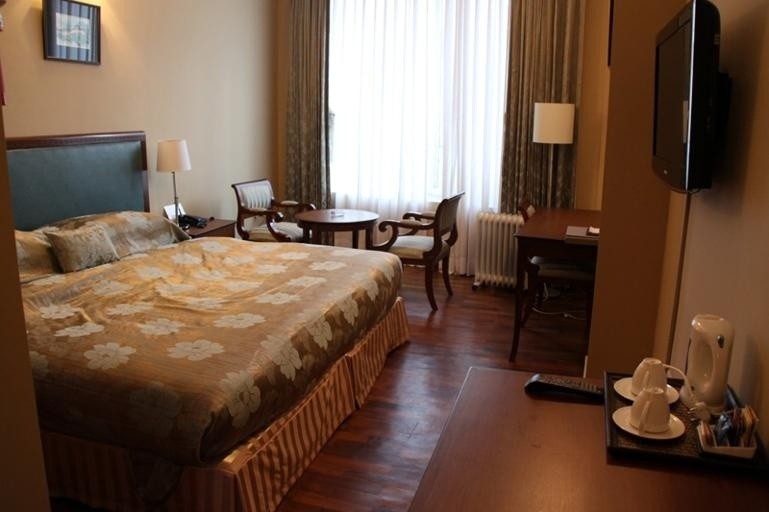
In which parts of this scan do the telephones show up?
[179,215,208,228]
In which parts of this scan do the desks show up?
[401,363,768,509]
[291,207,382,253]
[508,207,601,363]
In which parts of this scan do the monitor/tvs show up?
[651,0,721,190]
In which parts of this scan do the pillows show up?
[12,228,58,286]
[68,206,178,265]
[40,218,123,276]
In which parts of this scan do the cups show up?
[629,387,672,434]
[630,358,666,393]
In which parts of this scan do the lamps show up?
[154,137,195,232]
[529,102,576,208]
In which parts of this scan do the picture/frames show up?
[161,202,187,222]
[39,0,104,68]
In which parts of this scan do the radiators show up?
[472,210,530,294]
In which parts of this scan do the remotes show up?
[524,373,605,400]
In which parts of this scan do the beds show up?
[2,129,415,512]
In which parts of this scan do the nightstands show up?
[170,215,239,241]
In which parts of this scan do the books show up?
[565,225,600,238]
[586,226,600,235]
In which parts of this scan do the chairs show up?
[371,190,468,310]
[517,200,594,334]
[229,174,319,244]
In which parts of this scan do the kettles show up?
[681,312,735,417]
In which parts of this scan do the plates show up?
[613,376,680,404]
[612,407,685,440]
